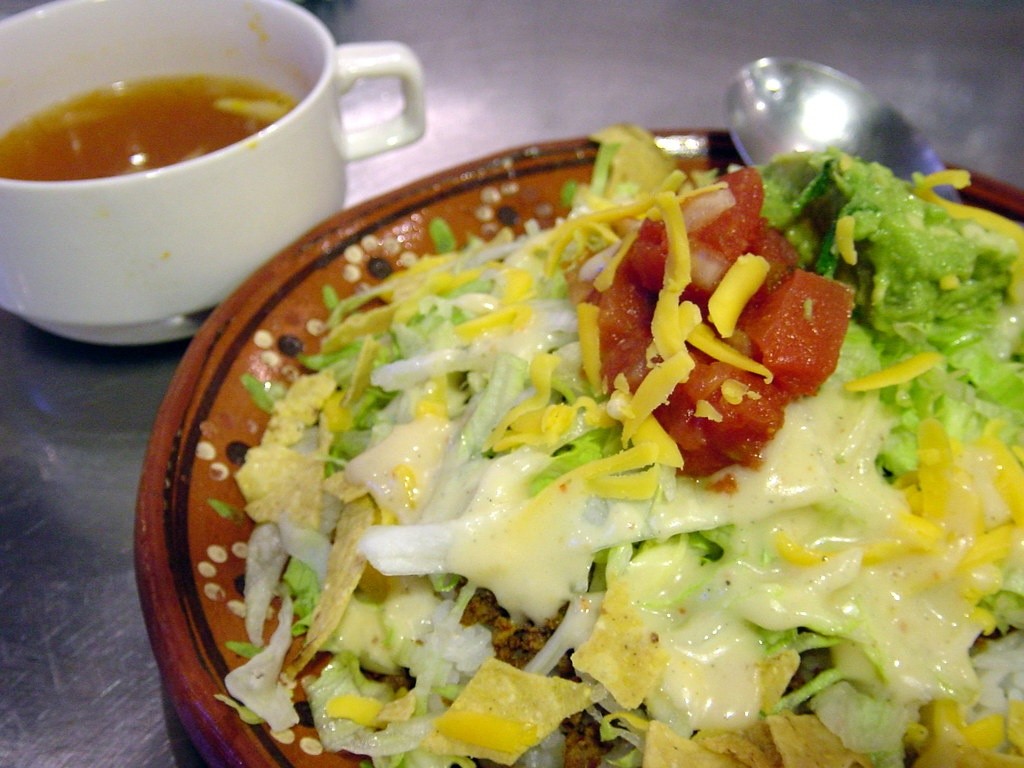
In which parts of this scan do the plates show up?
[133,119,1024,768]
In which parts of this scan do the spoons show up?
[724,54,962,205]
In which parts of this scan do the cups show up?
[0,0,427,346]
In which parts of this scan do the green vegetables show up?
[204,135,1023,768]
